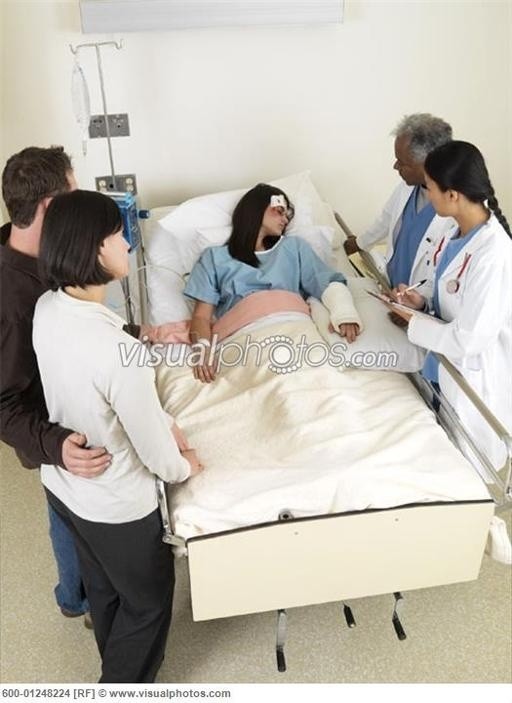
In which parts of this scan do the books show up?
[369,288,449,327]
[335,212,395,294]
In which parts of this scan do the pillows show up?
[155,173,341,278]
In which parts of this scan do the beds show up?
[134,169,510,670]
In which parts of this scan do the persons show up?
[345,109,453,409]
[387,138,511,490]
[2,141,158,632]
[180,180,367,385]
[33,187,208,682]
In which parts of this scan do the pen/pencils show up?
[396,278,427,296]
